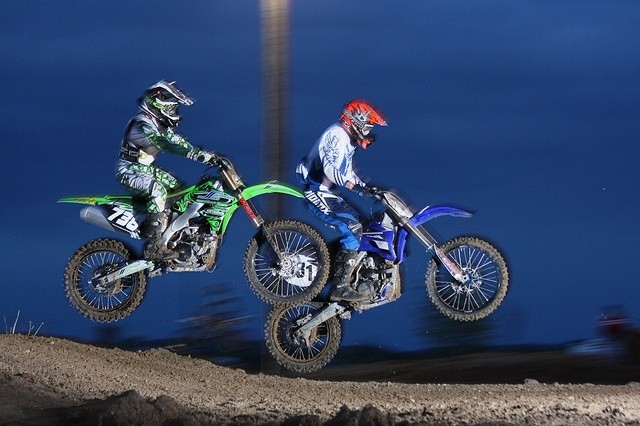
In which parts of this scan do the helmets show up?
[338,99,389,150]
[137,79,194,127]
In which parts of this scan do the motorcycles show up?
[264,184,509,374]
[57,150,330,324]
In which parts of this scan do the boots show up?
[144,212,180,260]
[330,250,369,302]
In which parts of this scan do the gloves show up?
[351,184,371,199]
[191,149,212,164]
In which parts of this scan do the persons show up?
[295,100,388,301]
[115,80,219,260]
